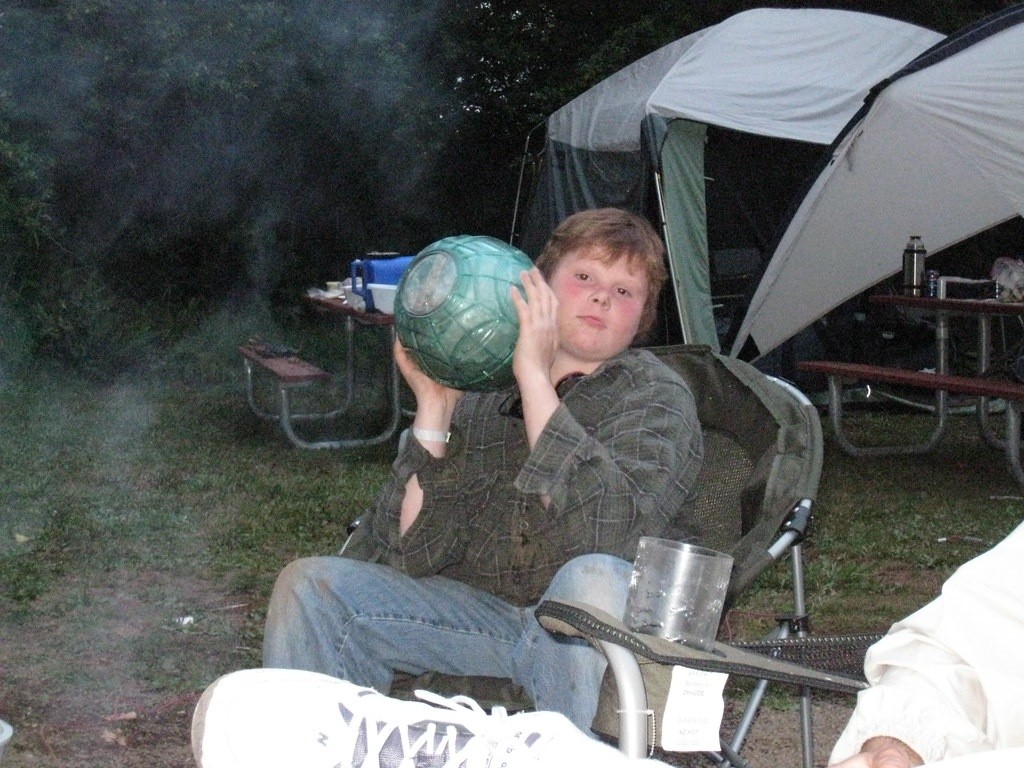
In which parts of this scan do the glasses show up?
[497,373,587,420]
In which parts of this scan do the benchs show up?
[237,339,325,383]
[797,359,1023,402]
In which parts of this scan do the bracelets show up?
[413,427,451,443]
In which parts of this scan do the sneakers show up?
[191,668,541,768]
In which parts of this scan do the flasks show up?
[900,236,927,298]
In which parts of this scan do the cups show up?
[623,536,734,654]
[327,282,341,293]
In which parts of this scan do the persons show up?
[190,521,1023,768]
[261,207,705,739]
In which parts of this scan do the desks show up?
[871,293,1024,453]
[303,289,406,441]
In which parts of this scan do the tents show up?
[510,0,1024,364]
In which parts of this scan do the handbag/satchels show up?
[351,256,418,313]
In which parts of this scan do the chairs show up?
[534,599,889,768]
[343,339,840,768]
